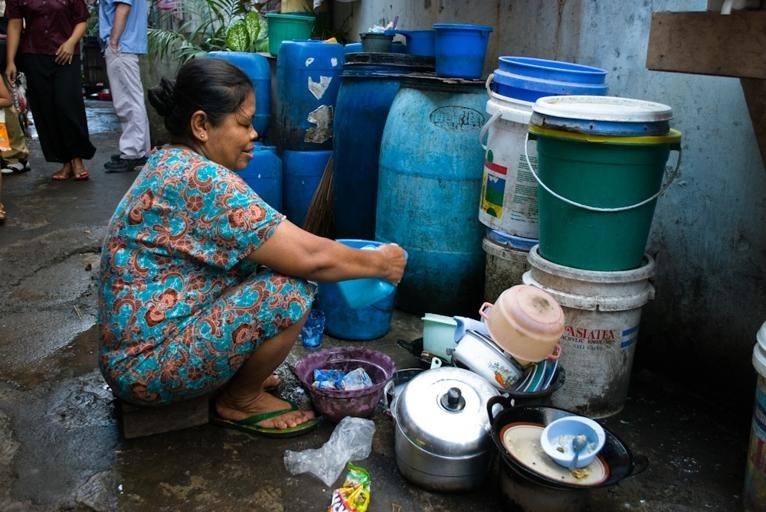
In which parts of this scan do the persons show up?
[0,74,14,107]
[3,1,97,180]
[94,56,409,442]
[93,0,152,174]
[0,0,31,175]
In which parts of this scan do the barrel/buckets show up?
[527,244,656,296]
[481,229,538,304]
[284,148,330,226]
[204,46,268,141]
[330,48,432,242]
[261,15,314,55]
[233,144,284,210]
[313,227,399,343]
[398,23,432,56]
[431,18,494,79]
[486,56,607,104]
[743,320,766,511]
[477,90,608,239]
[376,76,484,287]
[521,271,655,421]
[269,38,342,145]
[525,95,684,269]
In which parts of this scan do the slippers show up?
[210,398,320,438]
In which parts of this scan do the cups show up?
[302,309,326,347]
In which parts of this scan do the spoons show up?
[568,429,585,472]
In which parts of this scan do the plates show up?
[501,420,608,486]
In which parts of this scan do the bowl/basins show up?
[291,345,396,422]
[420,281,566,388]
[358,32,395,53]
[542,414,607,464]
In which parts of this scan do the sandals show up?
[1,158,31,175]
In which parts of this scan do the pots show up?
[398,337,426,362]
[382,366,499,491]
[483,391,644,488]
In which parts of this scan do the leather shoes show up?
[52,153,147,179]
